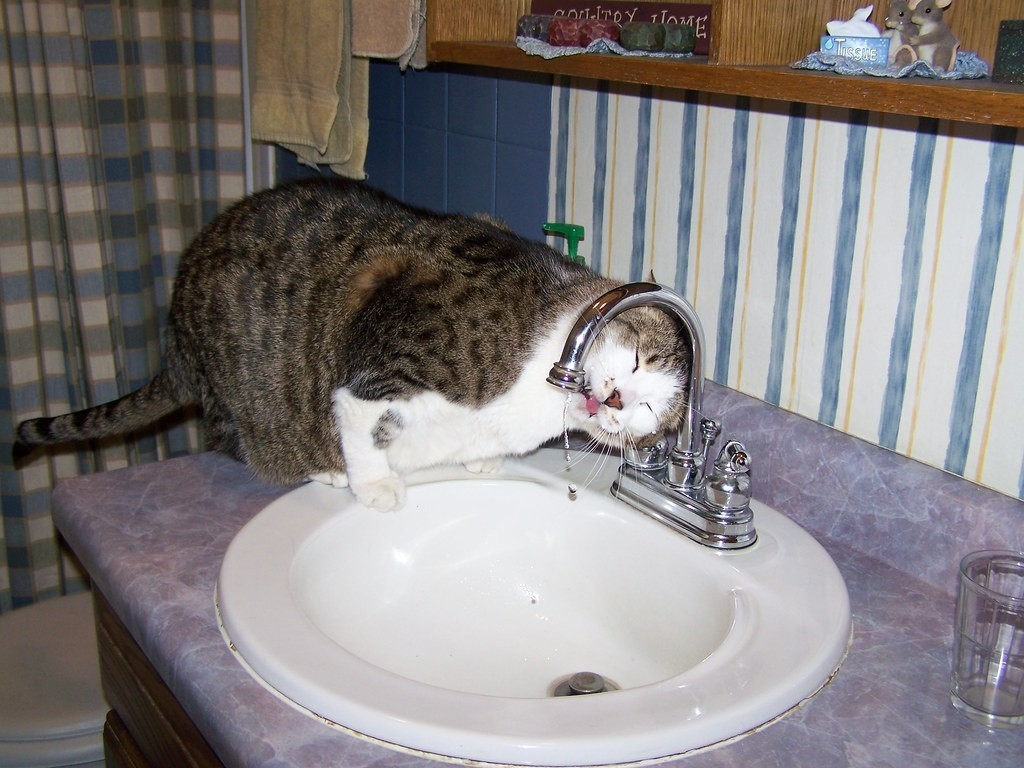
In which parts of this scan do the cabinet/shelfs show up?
[89,573,223,767]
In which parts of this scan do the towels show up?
[251,0,426,180]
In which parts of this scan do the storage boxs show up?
[821,35,890,67]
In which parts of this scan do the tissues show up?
[820,5,889,68]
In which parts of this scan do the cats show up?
[15,176,694,512]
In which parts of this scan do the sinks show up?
[217,448,851,767]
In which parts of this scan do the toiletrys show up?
[544,223,585,268]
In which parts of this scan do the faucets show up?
[547,282,705,488]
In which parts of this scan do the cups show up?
[950,550,1024,730]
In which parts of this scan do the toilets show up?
[0,592,108,768]
